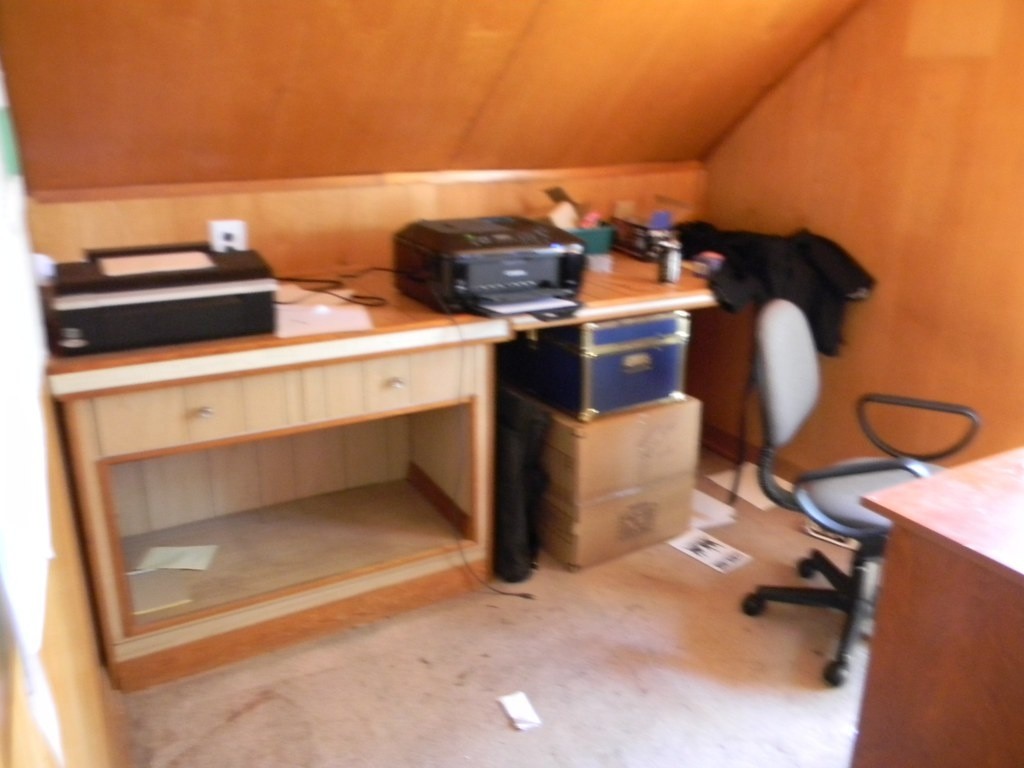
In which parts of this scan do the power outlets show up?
[211,220,245,253]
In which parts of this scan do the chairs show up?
[739,296,981,684]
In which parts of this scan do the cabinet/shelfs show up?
[849,444,1024,768]
[41,266,491,691]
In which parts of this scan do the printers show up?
[391,214,589,320]
[36,220,277,358]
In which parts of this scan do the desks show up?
[496,249,764,467]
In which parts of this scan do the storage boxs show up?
[41,241,279,357]
[611,195,689,262]
[567,222,616,253]
[495,389,703,571]
[503,309,692,421]
